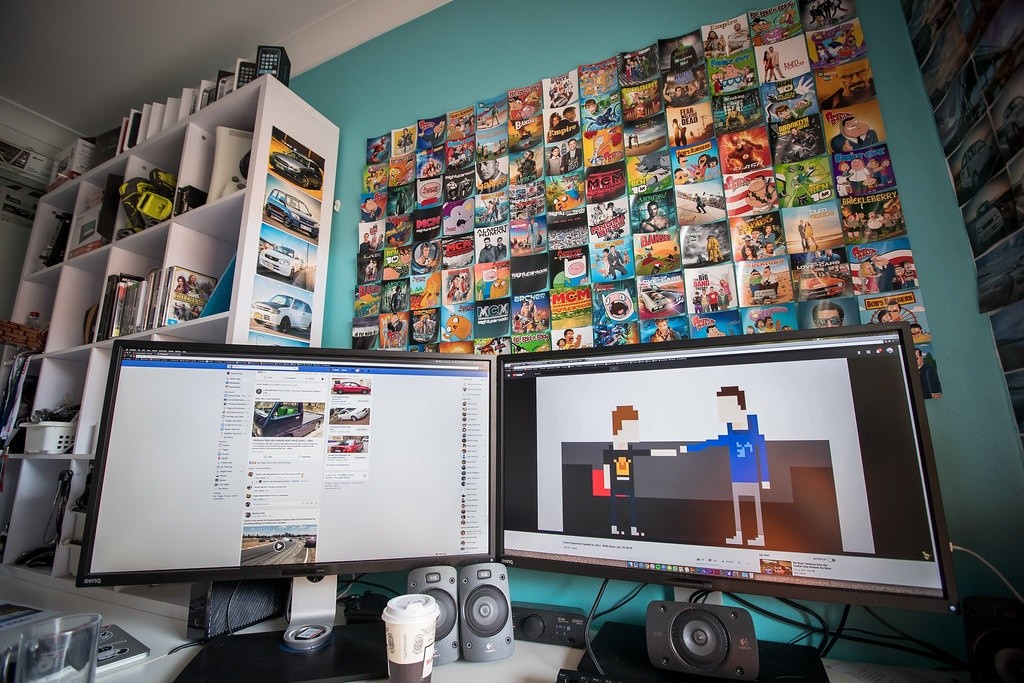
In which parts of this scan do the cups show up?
[0,614,103,683]
[382,594,439,683]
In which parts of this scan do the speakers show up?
[963,594,1024,683]
[646,600,759,680]
[407,566,460,666]
[458,562,514,662]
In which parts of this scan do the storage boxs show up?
[67,203,105,259]
[258,45,292,87]
[64,543,81,577]
[18,421,77,454]
[162,97,181,131]
[215,68,234,100]
[46,138,95,191]
[234,59,256,88]
[115,101,165,155]
[91,125,121,168]
[179,88,197,120]
[195,79,217,113]
[71,510,87,542]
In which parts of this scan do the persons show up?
[546,107,580,142]
[693,193,706,214]
[527,217,539,253]
[821,59,876,110]
[549,79,573,101]
[479,237,506,263]
[359,233,383,251]
[789,127,806,144]
[703,23,749,57]
[742,224,779,259]
[706,235,724,262]
[798,220,819,251]
[639,202,670,233]
[546,139,582,174]
[650,317,682,341]
[763,47,785,82]
[811,303,845,327]
[401,128,413,146]
[476,159,507,194]
[812,249,840,265]
[662,39,706,106]
[694,280,733,312]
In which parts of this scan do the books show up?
[97,266,217,341]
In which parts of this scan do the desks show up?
[109,606,971,683]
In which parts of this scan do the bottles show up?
[17,311,40,354]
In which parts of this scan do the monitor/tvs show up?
[75,339,498,683]
[495,320,961,683]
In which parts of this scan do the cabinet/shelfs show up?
[0,72,340,621]
